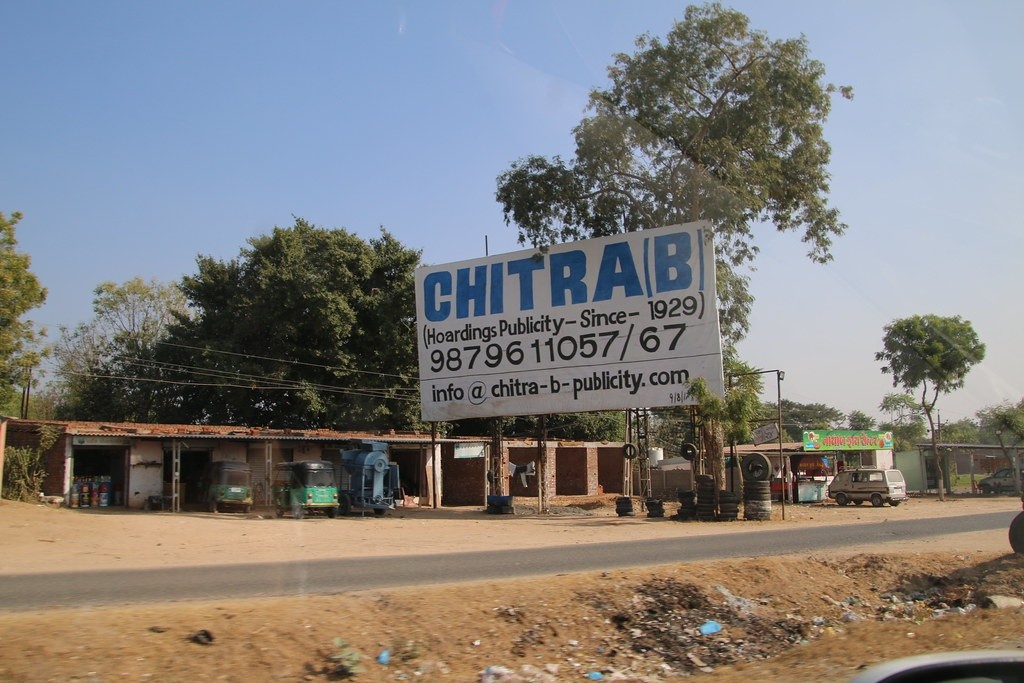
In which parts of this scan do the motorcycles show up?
[198,458,255,513]
[265,459,340,519]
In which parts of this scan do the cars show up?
[977,467,1024,495]
[850,647,1023,682]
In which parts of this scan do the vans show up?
[825,468,910,507]
